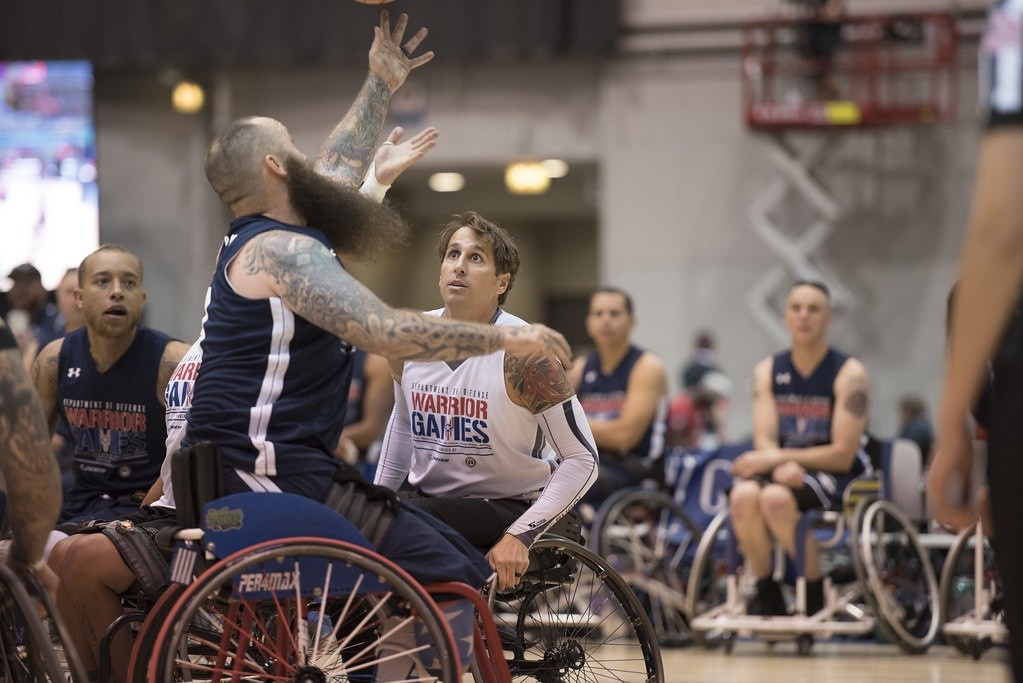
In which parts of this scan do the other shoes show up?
[804,579,824,616]
[745,578,788,616]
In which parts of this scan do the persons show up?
[170,8,495,679]
[24,239,207,682]
[51,122,439,683]
[723,273,875,628]
[24,262,88,425]
[887,390,937,475]
[0,259,56,458]
[368,210,603,681]
[633,387,731,582]
[0,269,62,683]
[548,284,670,499]
[680,325,729,450]
[918,1,1022,682]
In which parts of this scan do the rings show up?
[380,139,397,146]
[514,571,522,577]
[400,44,413,59]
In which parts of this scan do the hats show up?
[8,264,40,281]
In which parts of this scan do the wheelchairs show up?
[929,504,1010,661]
[676,460,941,658]
[104,442,517,682]
[557,483,721,648]
[466,533,666,683]
